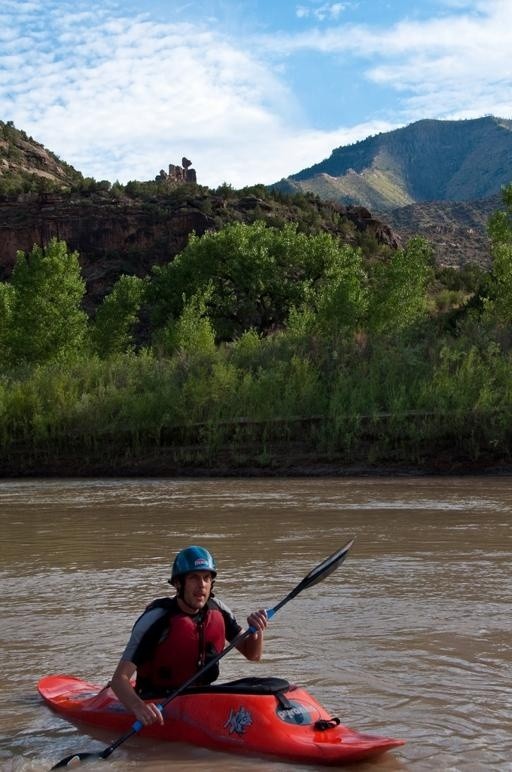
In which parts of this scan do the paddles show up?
[49,541,354,769]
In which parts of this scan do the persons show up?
[112,545,267,727]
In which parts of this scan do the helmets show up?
[168,546,218,585]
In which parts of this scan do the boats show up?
[38,672,404,765]
